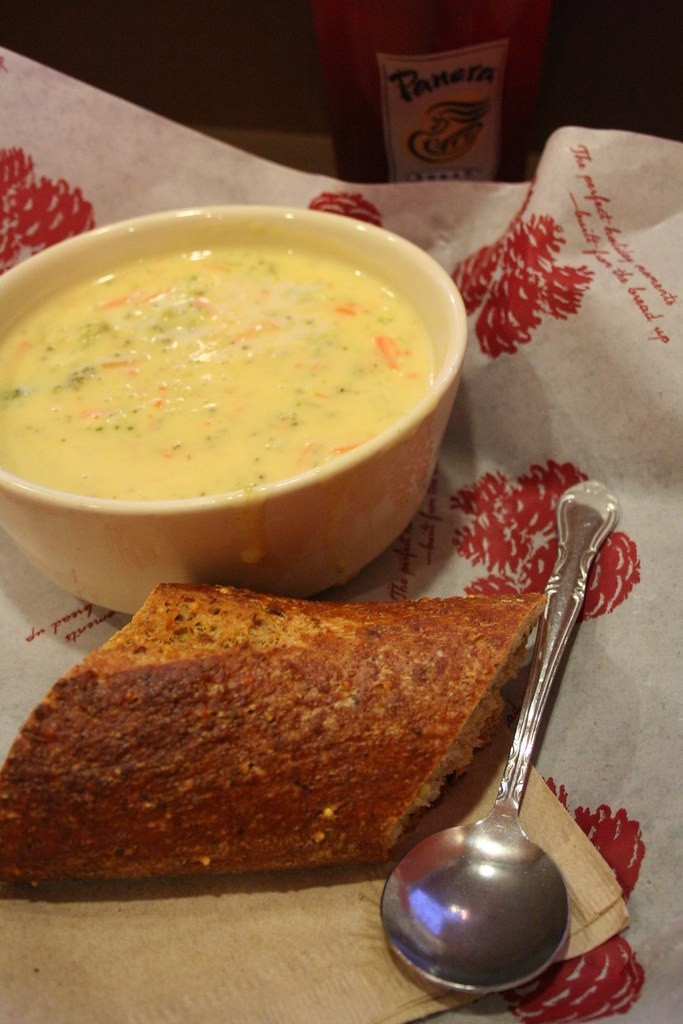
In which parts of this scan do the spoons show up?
[379,479,622,991]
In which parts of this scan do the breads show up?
[0,580,547,883]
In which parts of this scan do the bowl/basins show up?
[1,166,469,617]
